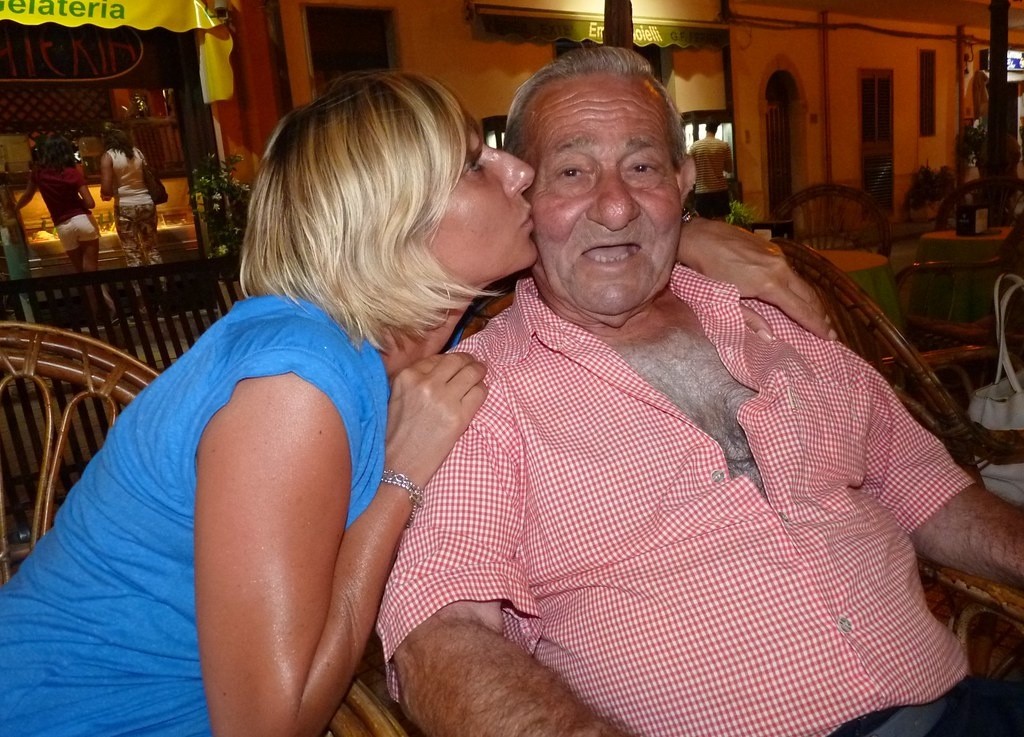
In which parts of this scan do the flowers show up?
[187,152,253,259]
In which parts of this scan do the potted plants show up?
[905,168,948,221]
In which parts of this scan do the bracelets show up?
[381,470,424,527]
[680,207,698,223]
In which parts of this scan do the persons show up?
[685,116,733,220]
[7,134,118,324]
[0,70,837,737]
[377,44,1024,737]
[99,122,167,321]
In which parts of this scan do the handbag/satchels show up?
[966,271,1024,508]
[136,150,168,203]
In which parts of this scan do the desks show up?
[909,226,1015,322]
[818,250,904,334]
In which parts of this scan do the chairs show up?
[935,175,1024,242]
[894,210,1024,392]
[773,236,1024,489]
[767,182,893,256]
[0,321,411,736]
[447,237,1024,680]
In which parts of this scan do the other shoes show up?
[127,306,150,326]
[157,307,179,321]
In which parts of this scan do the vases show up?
[217,280,244,323]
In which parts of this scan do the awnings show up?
[470,3,729,49]
